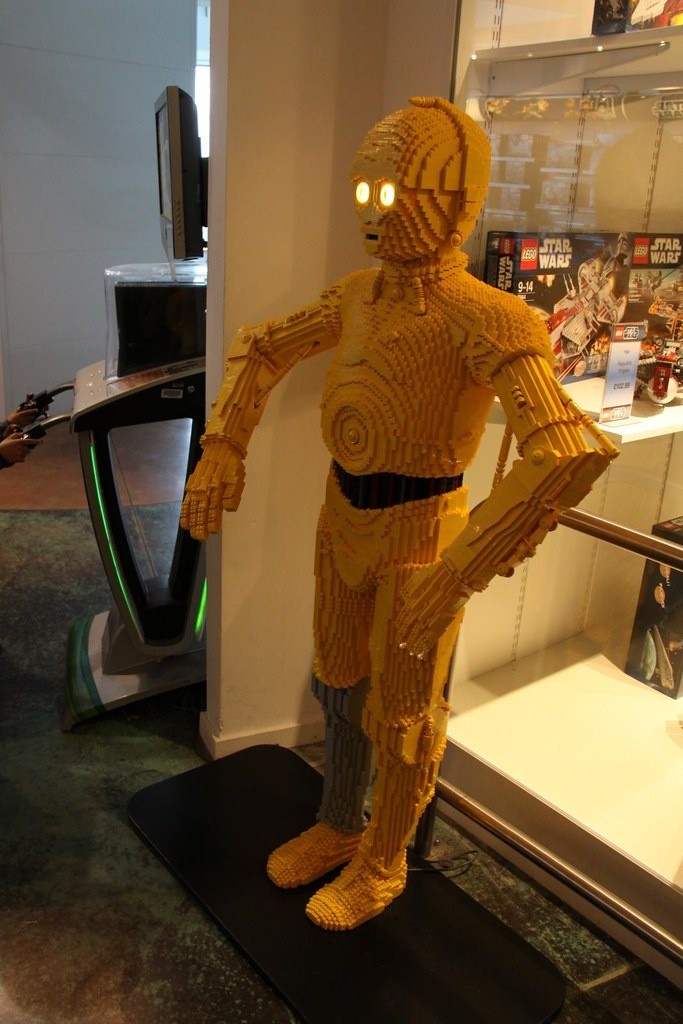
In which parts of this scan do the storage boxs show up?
[622,515,683,701]
[482,230,683,390]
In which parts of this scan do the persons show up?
[180,97,621,932]
[0,407,44,471]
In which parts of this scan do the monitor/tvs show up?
[155,85,205,262]
